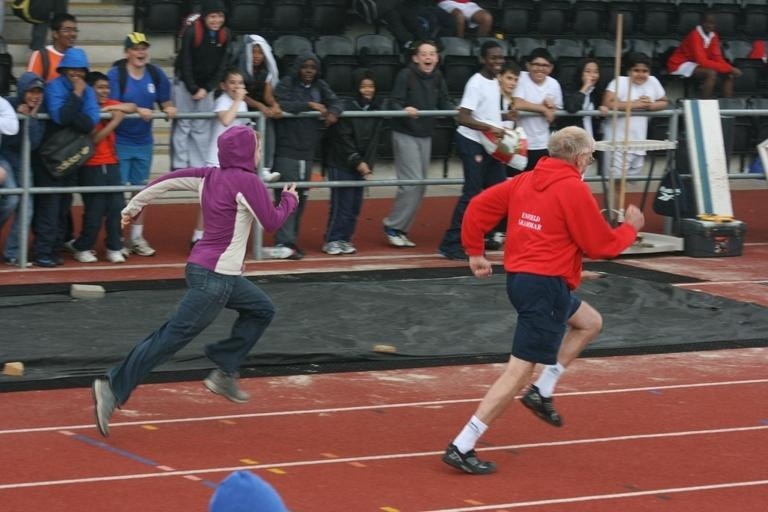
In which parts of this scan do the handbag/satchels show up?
[10,0,54,24]
[38,125,94,178]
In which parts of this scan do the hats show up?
[201,2,228,17]
[125,32,150,48]
[25,80,43,90]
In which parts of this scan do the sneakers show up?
[4,236,156,267]
[384,226,416,247]
[203,369,250,403]
[519,383,562,426]
[442,444,495,475]
[321,241,356,255]
[92,379,116,437]
[437,231,507,261]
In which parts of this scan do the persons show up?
[439,125,647,475]
[90,125,301,440]
[0,0,667,272]
[206,468,288,512]
[669,8,742,98]
